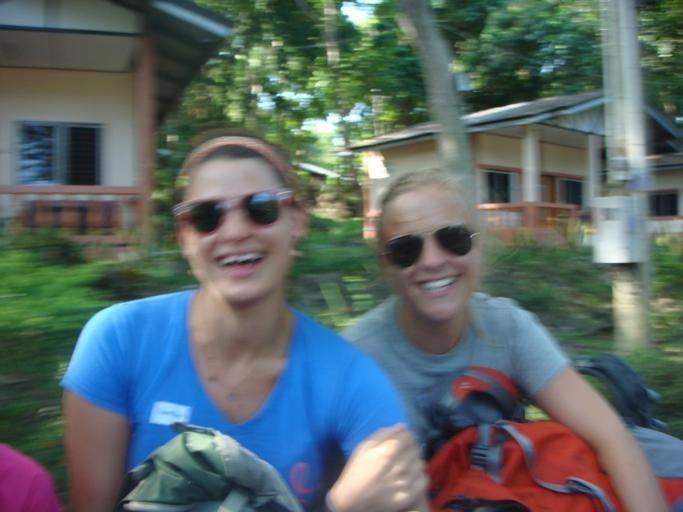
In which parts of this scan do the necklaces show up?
[187,317,286,409]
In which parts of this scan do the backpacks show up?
[424,353,682,512]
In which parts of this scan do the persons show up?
[309,165,672,512]
[0,440,64,510]
[54,125,435,512]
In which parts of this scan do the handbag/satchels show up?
[119,421,306,512]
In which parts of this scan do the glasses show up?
[377,224,480,266]
[173,190,296,235]
